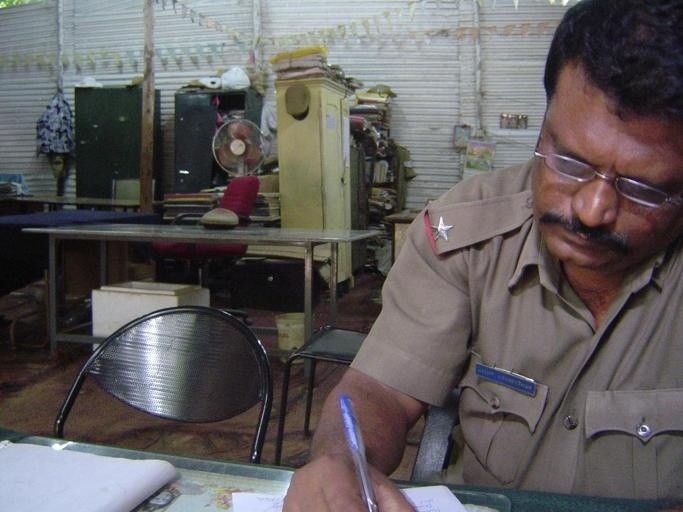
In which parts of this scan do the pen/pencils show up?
[339,396,377,512]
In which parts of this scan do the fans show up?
[212,118,271,180]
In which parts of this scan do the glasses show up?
[534,149,681,208]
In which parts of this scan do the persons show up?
[280,3,681,510]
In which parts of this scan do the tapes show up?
[207,78,221,89]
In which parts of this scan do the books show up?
[269,46,365,92]
[348,91,396,252]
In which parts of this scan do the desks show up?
[2,208,161,349]
[1,427,682,512]
[19,224,385,377]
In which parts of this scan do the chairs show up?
[154,176,262,325]
[49,301,274,462]
[111,178,155,200]
[275,325,372,463]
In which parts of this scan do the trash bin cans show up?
[275,312,304,364]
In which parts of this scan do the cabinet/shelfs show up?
[74,85,161,209]
[173,88,263,189]
[275,78,356,299]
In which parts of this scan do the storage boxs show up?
[91,280,210,354]
